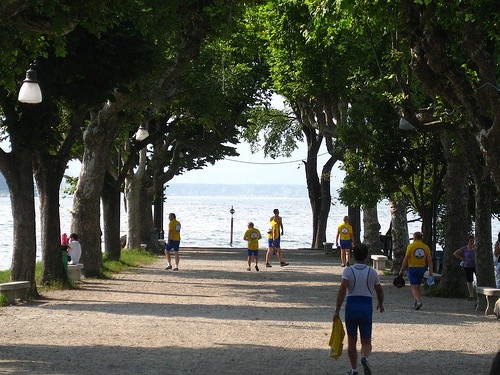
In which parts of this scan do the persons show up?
[243,222,262,271]
[332,242,388,375]
[61,233,82,265]
[453,235,477,302]
[335,216,356,267]
[269,208,284,255]
[164,213,182,271]
[266,214,289,267]
[398,231,434,310]
[493,232,500,290]
[385,220,394,261]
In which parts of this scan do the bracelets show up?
[400,268,405,271]
[334,308,339,312]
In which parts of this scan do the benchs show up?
[423,270,442,285]
[323,241,333,252]
[475,286,500,315]
[0,279,33,305]
[370,253,388,270]
[67,263,86,283]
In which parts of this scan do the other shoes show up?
[341,264,345,267]
[346,369,358,375]
[255,266,259,271]
[411,302,423,310]
[347,262,350,267]
[247,268,250,271]
[166,266,172,269]
[173,268,179,271]
[361,357,372,375]
[281,262,289,266]
[266,263,272,267]
